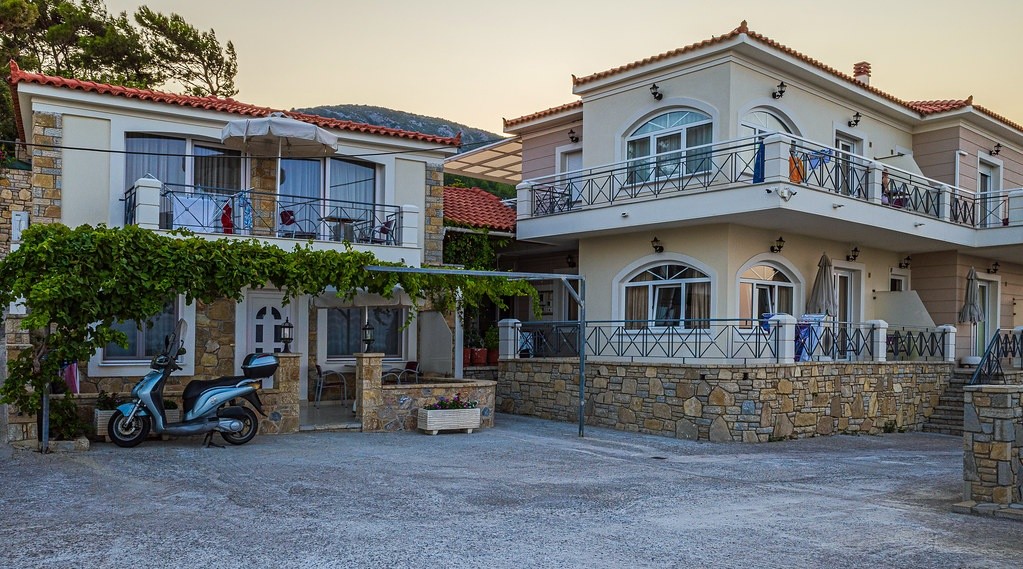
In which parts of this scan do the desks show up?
[345,364,392,411]
[318,217,365,242]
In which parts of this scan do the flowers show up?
[421,392,478,410]
[97,388,177,410]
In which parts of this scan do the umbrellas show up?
[957,264,986,358]
[220,115,339,231]
[806,251,838,359]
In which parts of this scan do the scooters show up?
[107,319,278,448]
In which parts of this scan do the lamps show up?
[650,236,664,253]
[986,261,1000,274]
[362,320,376,353]
[773,81,787,99]
[770,236,786,252]
[281,316,294,353]
[847,247,860,262]
[566,255,575,267]
[650,84,663,100]
[848,112,861,127]
[899,256,912,269]
[568,129,579,142]
[989,143,1002,155]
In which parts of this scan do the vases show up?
[417,407,481,435]
[94,408,180,443]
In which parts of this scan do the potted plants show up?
[461,325,499,368]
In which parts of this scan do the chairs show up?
[280,210,317,239]
[389,361,420,385]
[314,364,348,409]
[361,219,398,245]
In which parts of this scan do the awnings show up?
[309,280,455,309]
